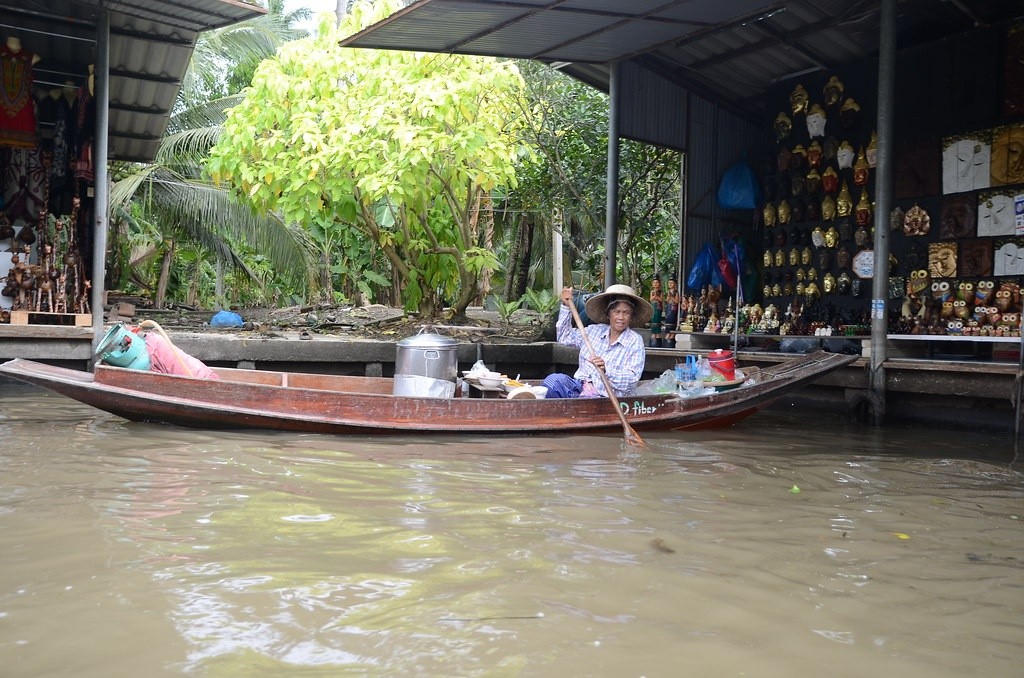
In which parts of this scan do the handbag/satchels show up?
[210,311,243,328]
[718,159,755,208]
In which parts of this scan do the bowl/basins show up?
[478,376,504,387]
[462,371,470,377]
[466,377,480,385]
[501,381,520,392]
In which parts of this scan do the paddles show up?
[562,286,647,447]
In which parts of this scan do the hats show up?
[585,284,654,329]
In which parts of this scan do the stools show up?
[463,377,504,398]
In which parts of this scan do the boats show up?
[0,349,860,436]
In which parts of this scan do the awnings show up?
[339,0,781,63]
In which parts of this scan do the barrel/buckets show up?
[706,349,739,380]
[394,325,460,386]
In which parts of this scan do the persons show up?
[0,36,96,223]
[650,273,663,338]
[541,284,653,398]
[763,76,878,297]
[664,274,679,339]
[681,294,806,335]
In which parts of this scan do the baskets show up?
[676,370,751,392]
[675,354,698,382]
